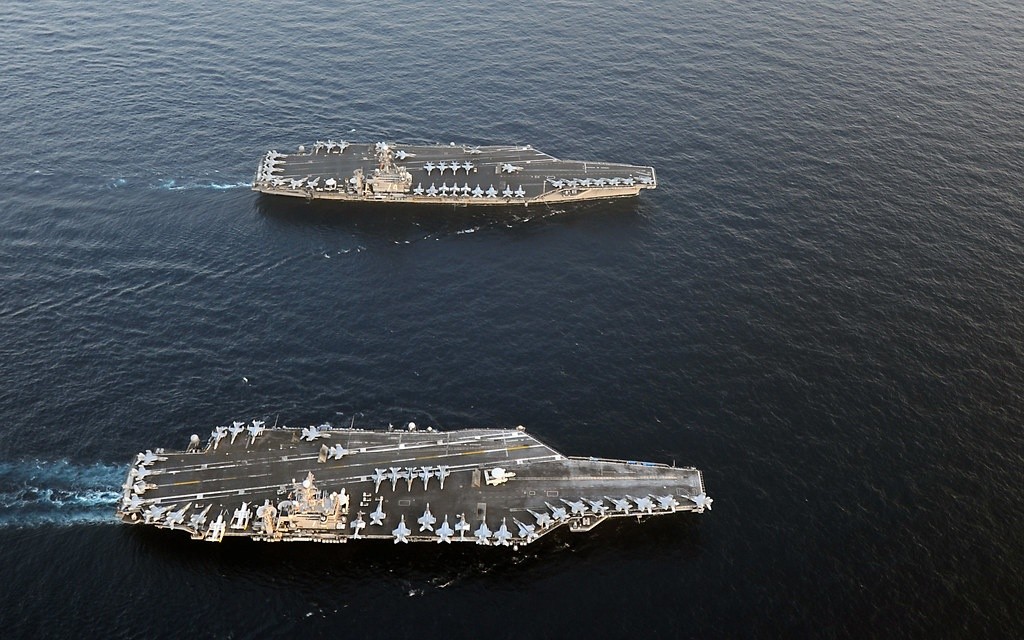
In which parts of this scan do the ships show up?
[118,417,713,553]
[250,143,657,209]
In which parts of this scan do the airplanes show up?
[461,147,482,155]
[299,426,331,443]
[325,443,351,463]
[120,449,225,546]
[501,161,522,175]
[328,485,349,510]
[412,182,528,200]
[546,176,655,188]
[423,159,473,177]
[211,419,265,450]
[231,500,276,531]
[261,149,320,193]
[526,490,713,526]
[373,141,417,161]
[314,139,348,155]
[351,463,539,549]
